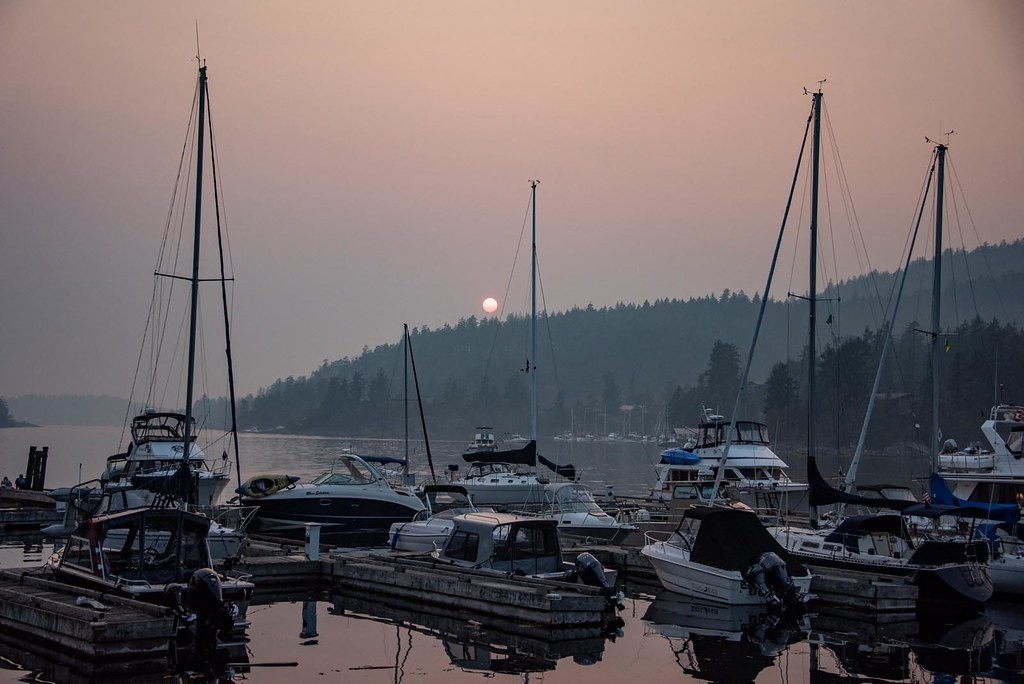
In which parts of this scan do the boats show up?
[637,499,814,607]
[328,509,632,642]
[238,447,431,544]
[648,400,794,503]
[639,590,803,657]
[49,506,256,652]
[217,88,1024,639]
[0,480,65,529]
[928,401,1024,483]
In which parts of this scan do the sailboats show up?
[65,28,259,571]
[805,131,1023,599]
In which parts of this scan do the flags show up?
[1015,410,1022,422]
[921,485,931,503]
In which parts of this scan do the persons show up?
[1,474,26,489]
